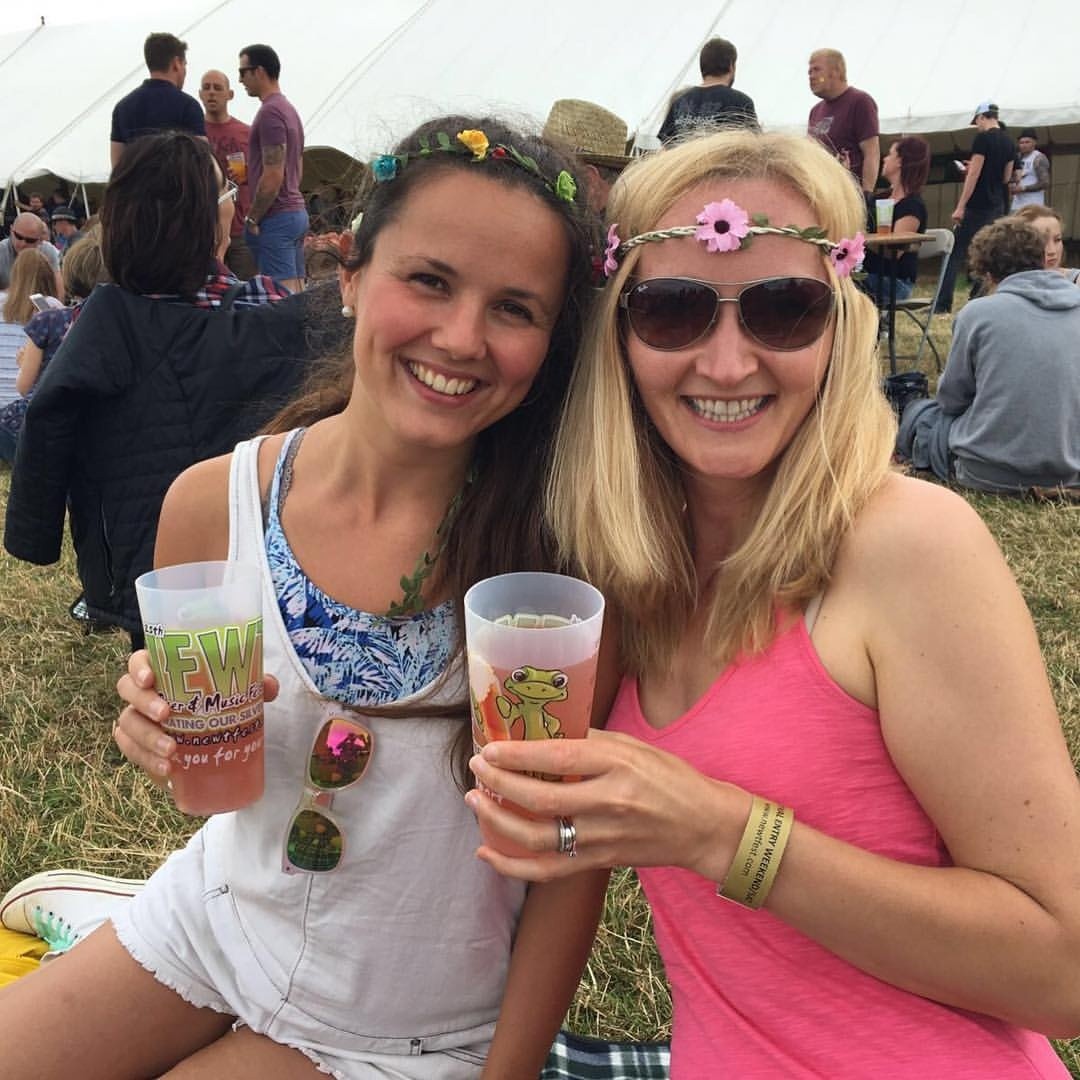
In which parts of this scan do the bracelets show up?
[716,795,796,912]
[245,217,257,225]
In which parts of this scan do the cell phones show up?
[28,293,50,312]
[954,160,967,172]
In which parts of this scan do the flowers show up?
[352,130,868,277]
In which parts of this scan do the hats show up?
[527,99,640,168]
[971,103,999,125]
[50,206,77,220]
[1017,130,1038,140]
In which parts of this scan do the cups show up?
[136,560,266,819]
[226,151,246,184]
[463,572,606,860]
[876,199,896,235]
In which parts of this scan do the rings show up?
[556,816,577,858]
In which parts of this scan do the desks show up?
[861,235,937,379]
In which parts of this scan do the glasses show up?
[13,227,40,243]
[239,67,260,77]
[282,716,374,876]
[619,277,837,351]
[218,179,239,204]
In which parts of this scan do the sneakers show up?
[0,869,149,961]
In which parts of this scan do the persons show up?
[0,112,609,1080]
[544,99,641,211]
[805,48,1079,492]
[463,126,1079,1080]
[0,30,363,473]
[656,39,765,152]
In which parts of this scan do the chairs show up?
[871,229,953,373]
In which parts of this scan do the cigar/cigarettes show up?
[952,223,958,232]
